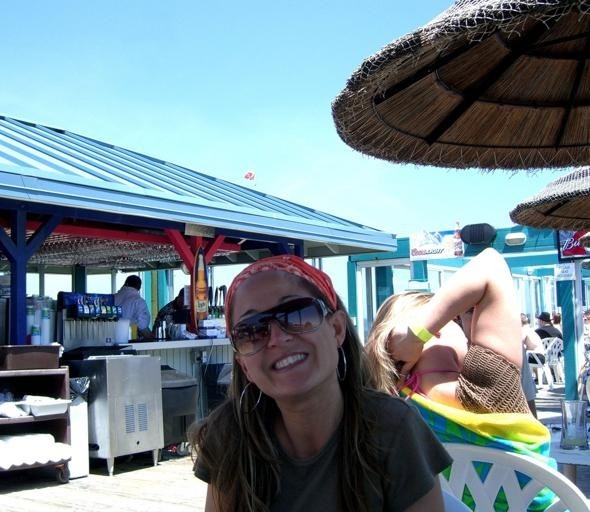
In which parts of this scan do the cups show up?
[155,327,163,340]
[131,324,138,339]
[560,400,589,449]
[160,320,167,339]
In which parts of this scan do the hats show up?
[536,312,553,322]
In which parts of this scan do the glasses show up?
[228,295,333,356]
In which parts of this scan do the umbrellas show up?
[508,168,589,231]
[329,0,589,169]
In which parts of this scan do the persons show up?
[151,288,191,335]
[520,309,589,486]
[363,248,567,512]
[187,256,453,511]
[114,275,151,338]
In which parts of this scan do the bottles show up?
[208,307,226,319]
[195,253,208,321]
[26,294,55,346]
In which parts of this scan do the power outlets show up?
[194,351,201,364]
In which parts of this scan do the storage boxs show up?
[0,344,59,370]
[29,399,71,416]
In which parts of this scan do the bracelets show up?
[406,318,434,342]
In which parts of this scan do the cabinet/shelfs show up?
[0,365,71,483]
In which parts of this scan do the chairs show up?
[437,336,590,512]
